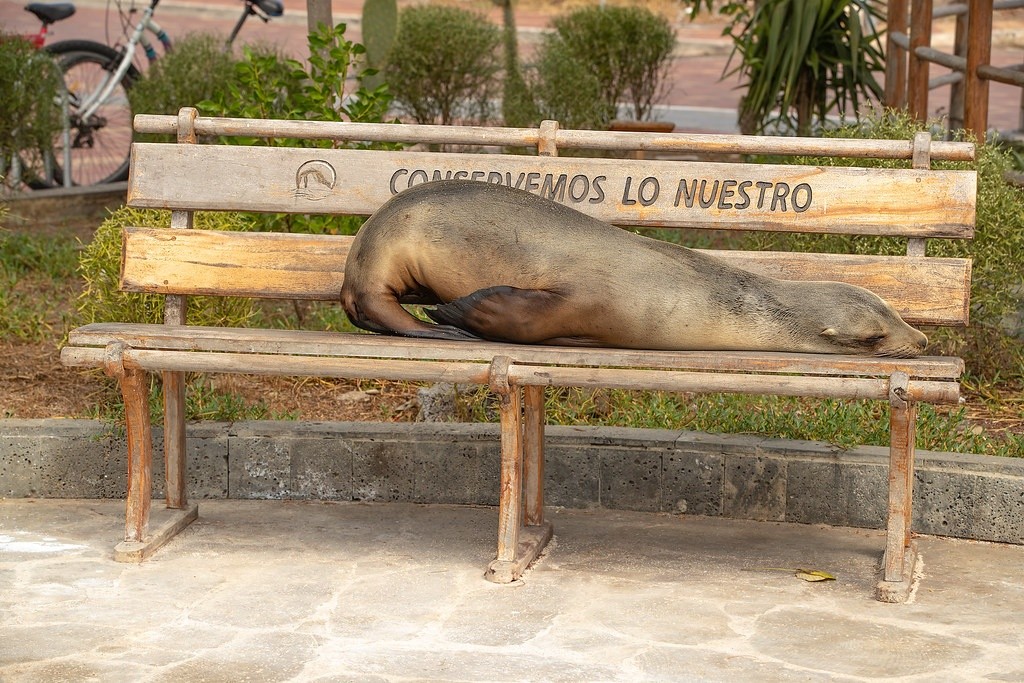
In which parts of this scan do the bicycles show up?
[37,0,285,186]
[0,0,147,189]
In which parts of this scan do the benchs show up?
[59,106,978,601]
